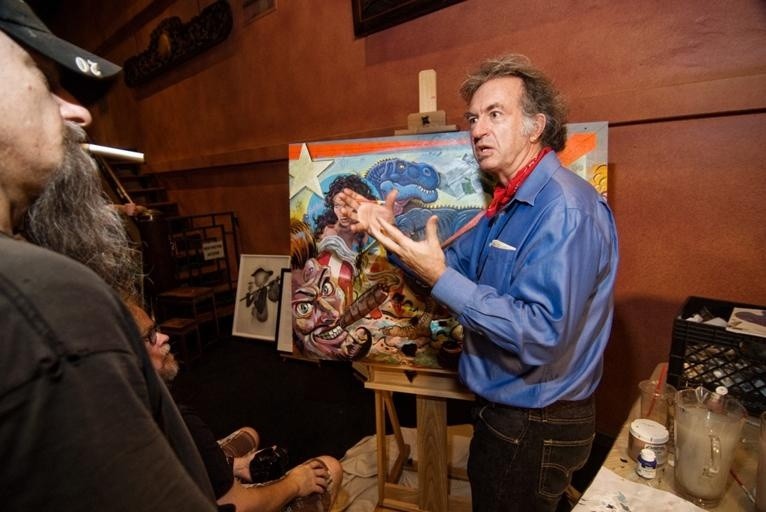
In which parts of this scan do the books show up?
[725,306,766,338]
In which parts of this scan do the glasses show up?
[140,323,162,346]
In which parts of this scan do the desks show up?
[569,362,766,512]
[158,286,221,348]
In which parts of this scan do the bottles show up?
[636,448,657,479]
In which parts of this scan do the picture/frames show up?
[238,0,277,28]
[352,0,467,38]
[232,254,295,354]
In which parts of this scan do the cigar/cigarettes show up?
[79,141,147,164]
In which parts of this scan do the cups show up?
[637,378,678,431]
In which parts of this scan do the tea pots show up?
[673,385,748,509]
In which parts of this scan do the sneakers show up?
[329,487,349,512]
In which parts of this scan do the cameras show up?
[249,443,304,483]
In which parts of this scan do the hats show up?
[0,0,121,105]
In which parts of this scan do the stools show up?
[161,318,201,363]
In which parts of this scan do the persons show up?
[124,298,343,512]
[313,175,377,251]
[337,52,626,510]
[0,2,221,512]
[290,216,372,360]
